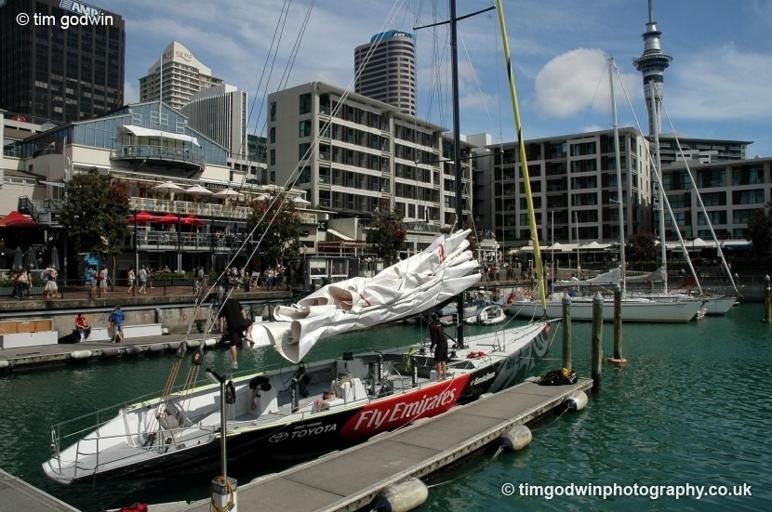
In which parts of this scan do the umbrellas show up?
[180,214,208,232]
[184,185,214,203]
[211,188,244,198]
[151,182,185,202]
[285,197,311,208]
[128,211,156,235]
[252,194,274,201]
[155,213,185,231]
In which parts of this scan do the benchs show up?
[0,319,59,350]
[72,323,164,341]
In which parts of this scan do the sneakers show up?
[244,336,255,344]
[232,364,238,369]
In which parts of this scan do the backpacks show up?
[538,368,577,386]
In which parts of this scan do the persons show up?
[108,306,118,341]
[428,314,449,381]
[111,307,125,344]
[216,295,255,370]
[569,273,579,281]
[11,263,288,300]
[74,312,87,345]
[559,286,571,299]
